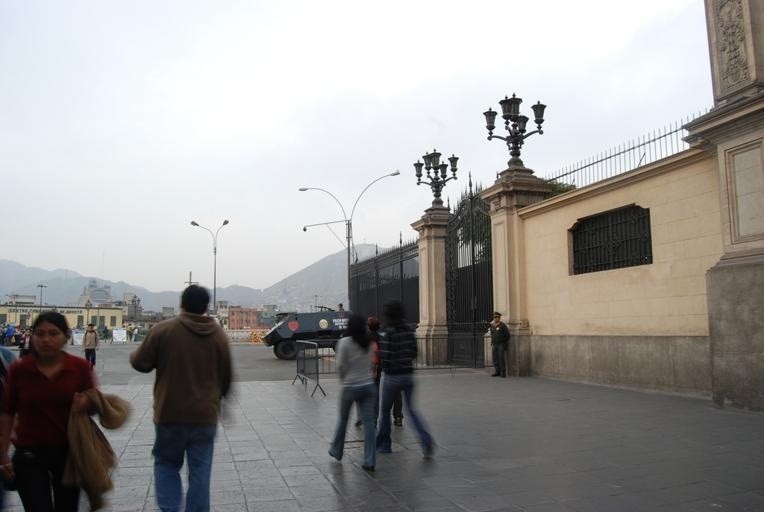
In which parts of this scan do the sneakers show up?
[394,417,402,426]
[328,451,342,460]
[362,464,373,471]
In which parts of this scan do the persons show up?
[488,312,510,377]
[125,284,234,512]
[0,322,32,358]
[1,311,100,511]
[391,387,404,428]
[329,313,378,472]
[351,315,380,427]
[80,322,99,370]
[377,298,436,461]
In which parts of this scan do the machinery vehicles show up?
[260,309,352,361]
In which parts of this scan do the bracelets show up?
[1,461,13,468]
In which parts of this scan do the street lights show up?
[483,92,546,166]
[36,284,47,315]
[84,299,92,325]
[131,294,139,319]
[297,169,401,303]
[190,218,229,314]
[411,149,458,205]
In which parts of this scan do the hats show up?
[493,312,501,317]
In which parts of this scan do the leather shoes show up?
[492,373,506,377]
